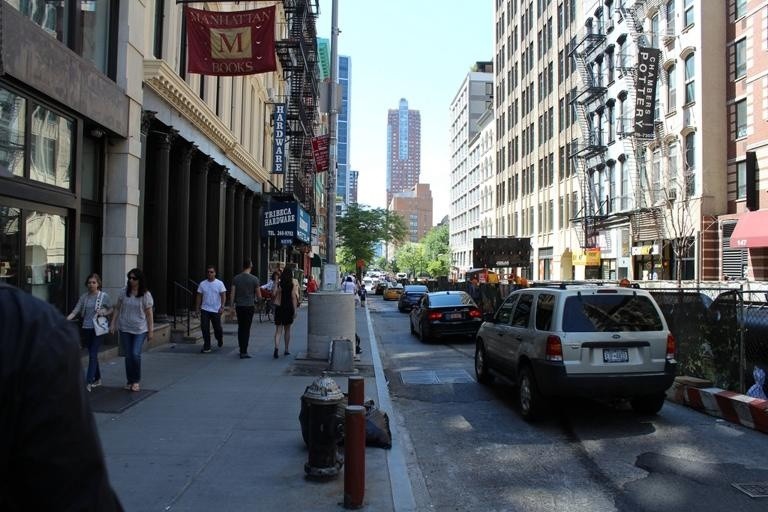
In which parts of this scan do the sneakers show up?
[86,378,141,393]
[201,343,290,359]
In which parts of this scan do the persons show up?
[272,266,297,358]
[0,281,122,512]
[307,275,319,293]
[66,274,113,391]
[110,268,154,391]
[196,265,227,353]
[230,260,262,358]
[260,272,280,314]
[340,274,368,308]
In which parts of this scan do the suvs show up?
[474,273,680,422]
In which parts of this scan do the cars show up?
[359,268,433,313]
[408,290,485,343]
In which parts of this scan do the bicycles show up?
[256,291,278,323]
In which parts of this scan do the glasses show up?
[129,276,138,281]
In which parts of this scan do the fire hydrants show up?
[298,371,345,481]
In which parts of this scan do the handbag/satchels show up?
[92,292,109,336]
[271,287,282,306]
